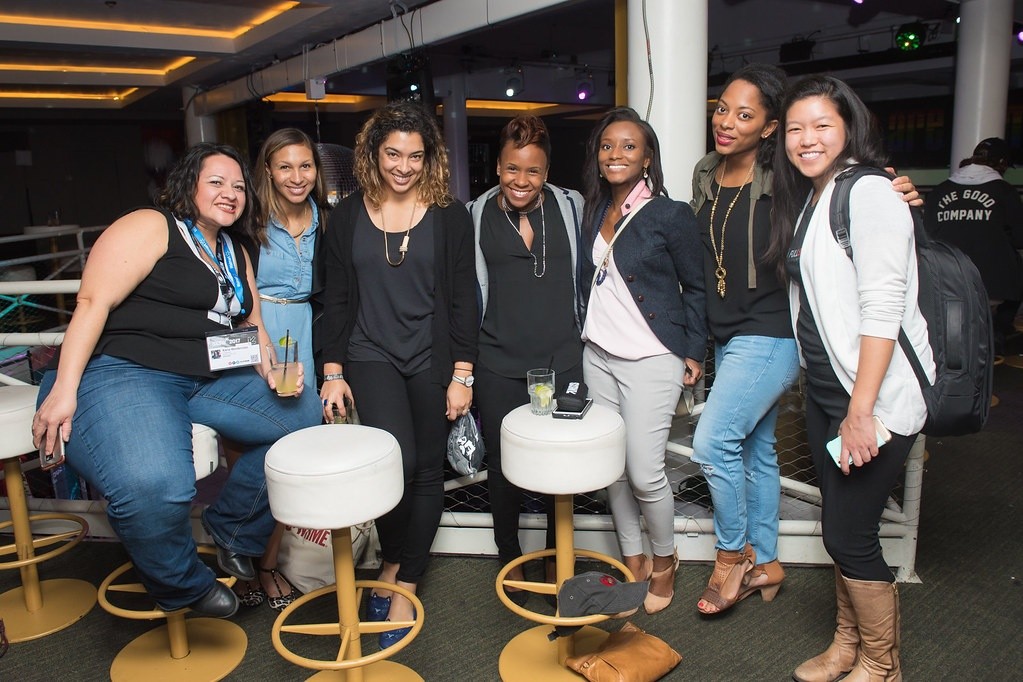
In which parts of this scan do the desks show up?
[24,223,81,330]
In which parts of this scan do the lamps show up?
[502,65,526,97]
[573,66,598,100]
[776,35,818,63]
[891,22,932,53]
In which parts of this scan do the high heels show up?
[736,559,785,603]
[609,555,654,619]
[643,546,678,615]
[696,542,756,619]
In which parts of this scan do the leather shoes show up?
[186,580,240,618]
[199,511,256,581]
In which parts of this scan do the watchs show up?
[453,374,476,388]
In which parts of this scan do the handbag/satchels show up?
[447,412,486,482]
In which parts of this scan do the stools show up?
[0,264,47,332]
[98,419,249,682]
[265,424,427,682]
[990,300,1005,409]
[0,385,98,642]
[496,396,638,682]
[61,254,87,302]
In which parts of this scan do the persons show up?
[31,141,323,618]
[576,104,703,618]
[688,61,926,616]
[314,102,484,653]
[772,74,939,681]
[221,128,333,613]
[921,138,1022,349]
[466,111,588,612]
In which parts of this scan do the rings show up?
[691,375,697,380]
[321,399,329,406]
[463,407,471,414]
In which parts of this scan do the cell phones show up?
[550,396,594,419]
[36,427,64,472]
[826,414,893,472]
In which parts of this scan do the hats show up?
[973,136,1018,171]
[548,571,650,642]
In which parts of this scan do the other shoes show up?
[257,566,302,617]
[501,561,529,607]
[232,581,264,609]
[542,556,557,609]
[366,587,391,622]
[378,609,417,650]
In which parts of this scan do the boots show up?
[840,573,903,682]
[792,565,861,682]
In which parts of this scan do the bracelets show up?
[454,367,475,374]
[323,373,344,381]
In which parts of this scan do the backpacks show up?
[829,163,992,437]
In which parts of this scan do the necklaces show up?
[369,186,423,266]
[593,196,627,286]
[501,188,552,278]
[702,156,769,300]
[270,198,320,242]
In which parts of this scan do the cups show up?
[328,399,353,425]
[527,368,556,417]
[265,340,300,396]
